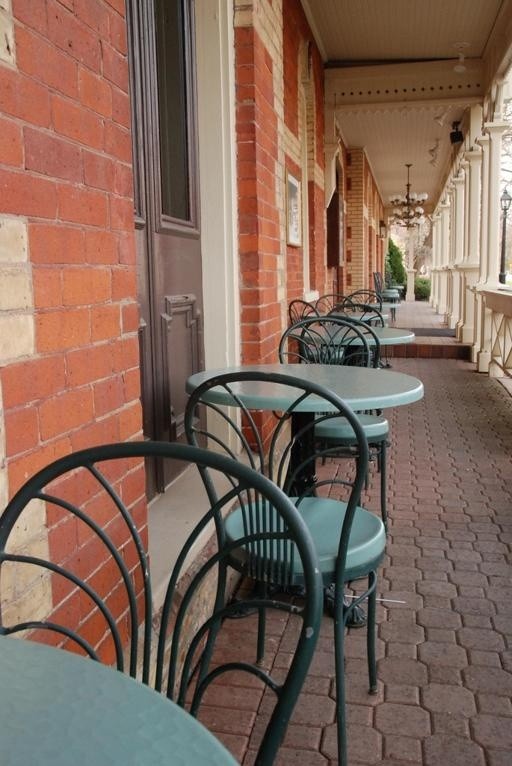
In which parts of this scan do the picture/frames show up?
[284,166,303,250]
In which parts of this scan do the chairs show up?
[275,272,407,536]
[3,438,324,762]
[183,371,387,766]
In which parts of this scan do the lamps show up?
[428,50,469,167]
[392,164,427,230]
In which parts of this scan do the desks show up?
[188,363,424,628]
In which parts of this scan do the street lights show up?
[497,186,512,285]
